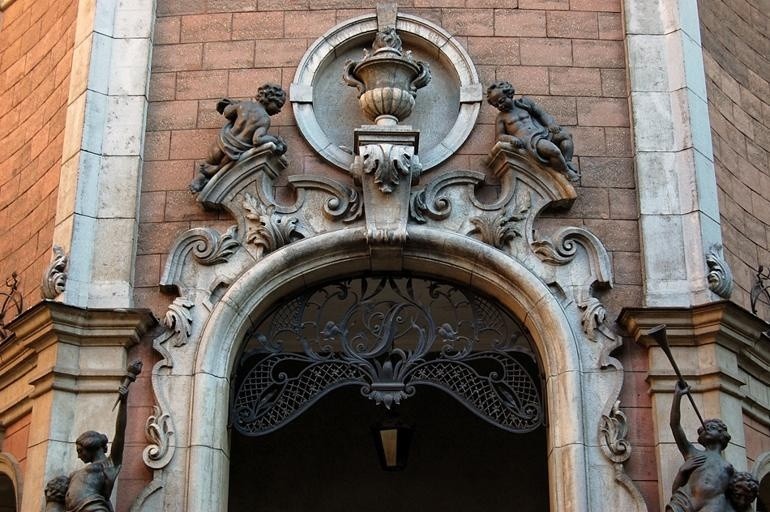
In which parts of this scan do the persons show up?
[664,380,735,512]
[671,454,761,512]
[44,474,70,512]
[187,82,286,195]
[486,80,582,182]
[63,385,130,512]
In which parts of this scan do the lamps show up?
[371,405,414,472]
